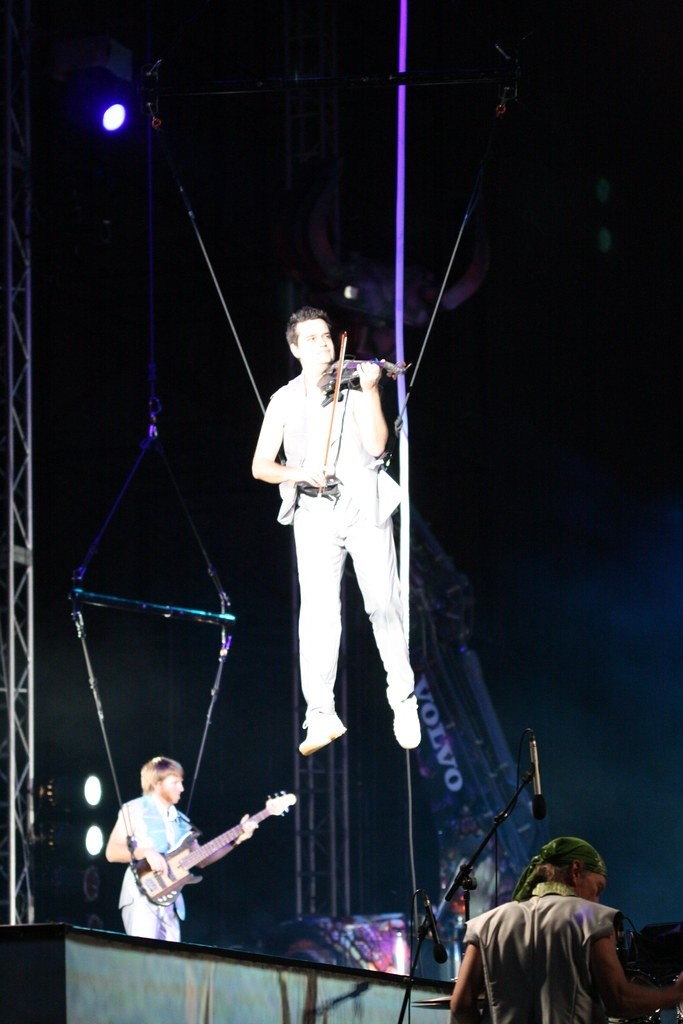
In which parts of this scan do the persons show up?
[449,837,682,1024]
[104,756,260,942]
[250,306,421,756]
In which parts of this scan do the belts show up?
[297,484,338,497]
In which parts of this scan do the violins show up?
[317,354,415,395]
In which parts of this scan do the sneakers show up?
[386,686,422,750]
[298,711,348,756]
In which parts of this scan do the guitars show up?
[133,789,299,907]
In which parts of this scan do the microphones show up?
[529,733,546,822]
[422,889,448,964]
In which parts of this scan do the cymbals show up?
[412,995,486,1010]
